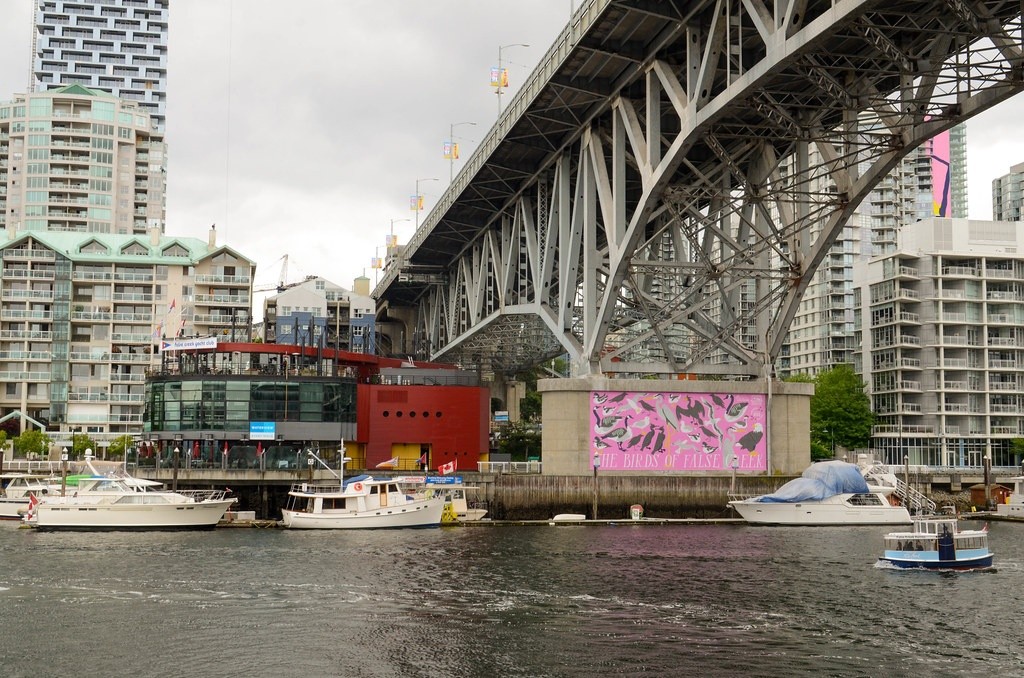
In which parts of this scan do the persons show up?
[896,541,924,551]
[140,442,154,458]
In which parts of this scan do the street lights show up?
[71,425,77,459]
[822,425,833,460]
[449,121,477,184]
[415,178,440,231]
[390,219,410,269]
[498,43,530,118]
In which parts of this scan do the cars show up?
[161,455,181,468]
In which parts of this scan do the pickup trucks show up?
[192,459,220,468]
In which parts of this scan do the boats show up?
[24,449,238,530]
[726,458,915,525]
[0,472,62,517]
[878,519,994,570]
[282,437,488,530]
[554,514,586,521]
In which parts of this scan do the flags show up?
[168,299,175,314]
[376,456,399,468]
[256,442,261,460]
[151,318,164,337]
[416,453,426,463]
[28,492,40,520]
[224,442,228,455]
[438,459,457,475]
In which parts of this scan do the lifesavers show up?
[354,483,362,491]
